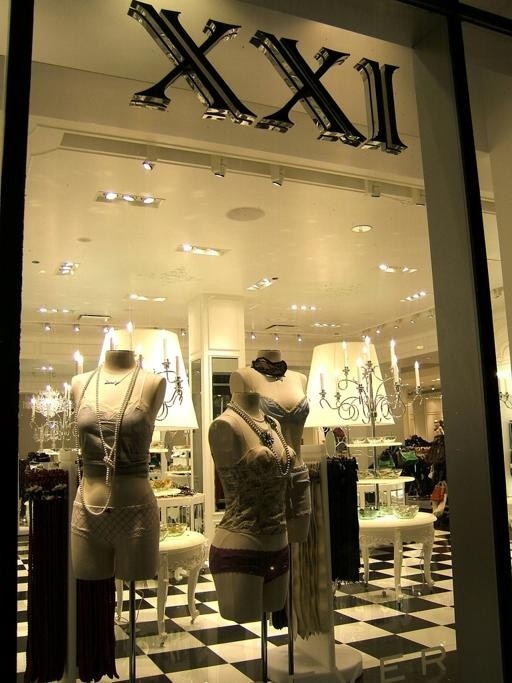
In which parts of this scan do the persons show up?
[204,388,295,625]
[228,347,310,544]
[433,419,444,437]
[68,348,168,580]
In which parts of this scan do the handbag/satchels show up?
[376,434,446,519]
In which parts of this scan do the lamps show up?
[29,320,199,430]
[497,373,512,410]
[318,335,420,510]
[300,341,396,458]
[58,132,426,207]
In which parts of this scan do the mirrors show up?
[208,354,240,516]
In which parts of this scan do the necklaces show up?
[226,402,292,478]
[249,356,288,378]
[73,363,143,517]
[102,359,140,385]
[226,400,266,422]
[95,362,139,486]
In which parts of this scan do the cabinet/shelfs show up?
[113,445,210,643]
[344,439,437,605]
